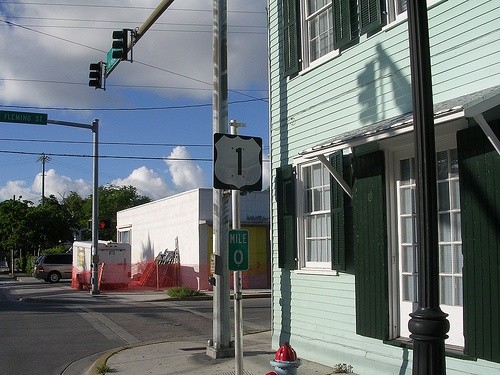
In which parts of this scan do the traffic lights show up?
[99,220,112,229]
[89,60,107,91]
[112,27,135,62]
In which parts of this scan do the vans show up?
[33,252,72,283]
